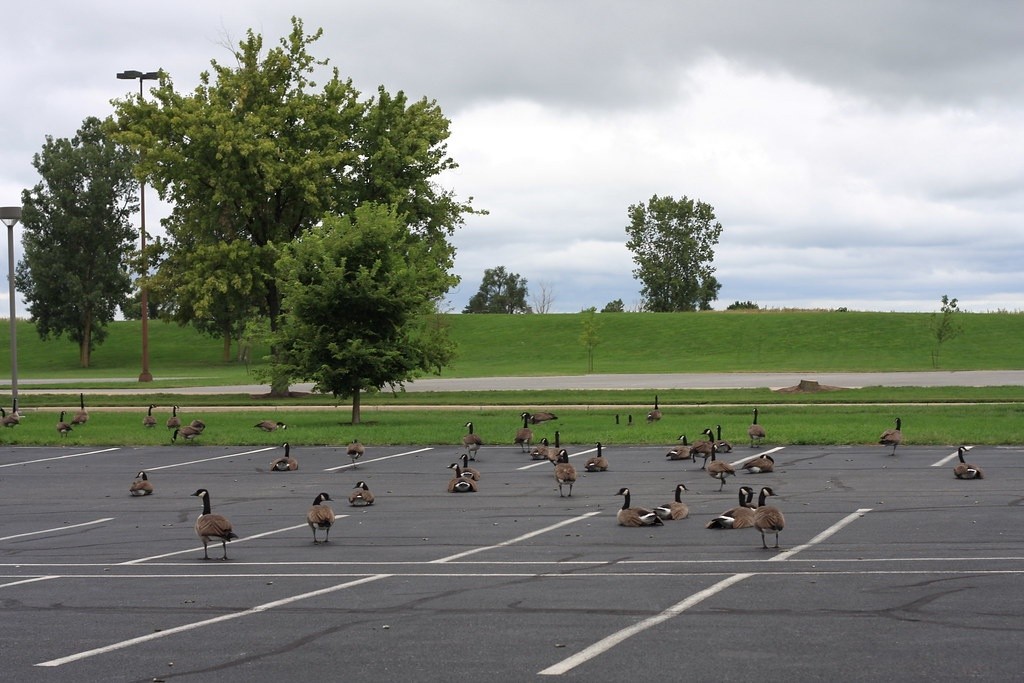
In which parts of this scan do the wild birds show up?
[514,412,608,497]
[167,405,181,431]
[954,446,984,480]
[254,421,288,432]
[613,406,784,552]
[348,480,375,507]
[190,489,239,559]
[270,442,299,472]
[56,411,73,437]
[0,398,20,427]
[142,404,156,428]
[129,471,153,496]
[445,421,484,492]
[190,420,205,432]
[878,417,902,456]
[614,394,662,426]
[71,393,89,426]
[171,425,201,444]
[306,492,336,543]
[346,439,365,469]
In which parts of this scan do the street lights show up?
[116,70,164,383]
[0,207,22,412]
